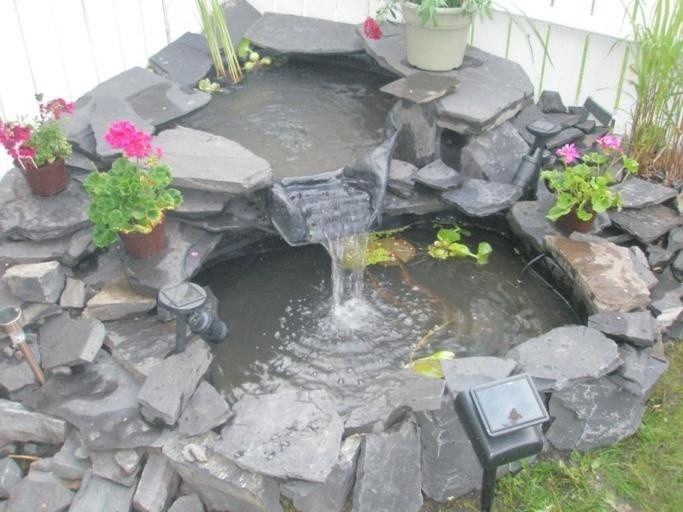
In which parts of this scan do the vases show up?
[558,204,599,236]
[12,159,68,198]
[117,213,167,258]
[402,0,473,72]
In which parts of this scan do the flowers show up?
[540,135,640,223]
[83,119,183,248]
[0,91,79,170]
[363,0,494,45]
